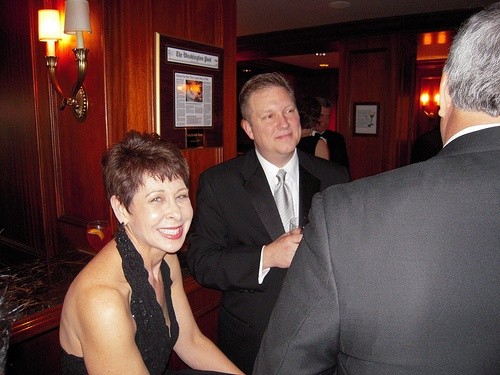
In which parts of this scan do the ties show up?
[273,169,298,233]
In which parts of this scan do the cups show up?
[87,220,112,251]
[290,217,308,236]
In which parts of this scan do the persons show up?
[246,8,500,375]
[290,92,331,161]
[306,94,352,184]
[56,129,246,375]
[410,125,443,165]
[182,73,352,375]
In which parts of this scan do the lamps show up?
[37,0,92,123]
[419,92,440,125]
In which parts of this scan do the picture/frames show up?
[153,32,223,150]
[352,101,380,137]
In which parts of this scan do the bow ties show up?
[315,133,328,139]
